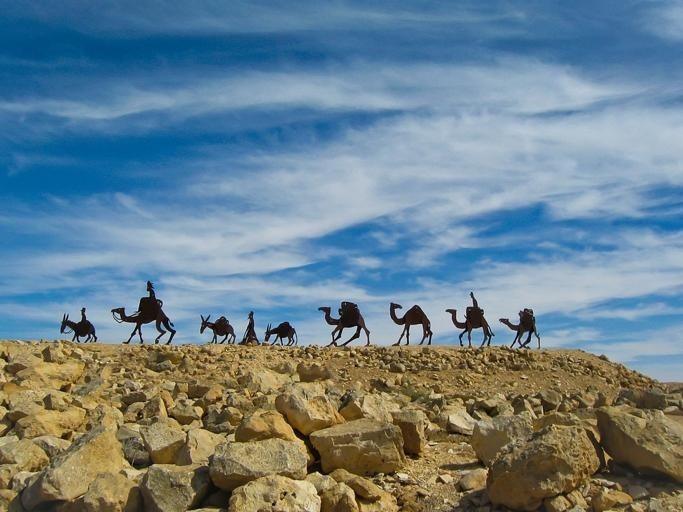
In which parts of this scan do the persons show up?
[77,307,86,324]
[469,291,477,308]
[243,311,260,345]
[145,281,155,301]
